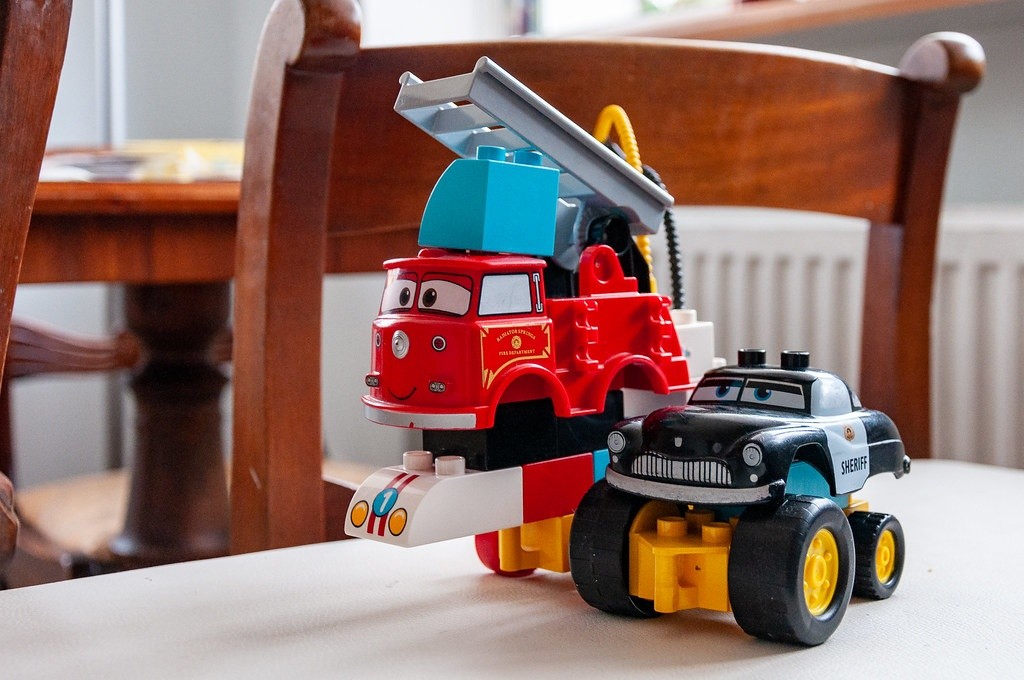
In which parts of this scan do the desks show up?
[18,138,423,578]
[0,462,1024,680]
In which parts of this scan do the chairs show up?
[230,0,985,557]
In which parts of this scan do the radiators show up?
[680,225,1024,469]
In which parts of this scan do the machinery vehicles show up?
[568,346,913,647]
[342,54,727,580]
